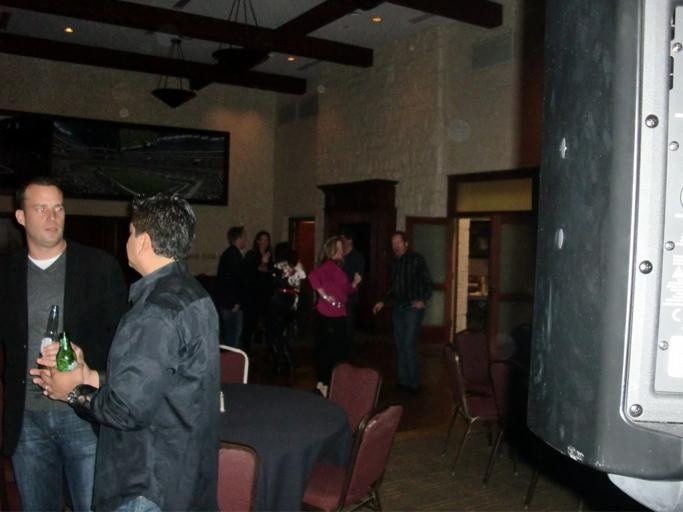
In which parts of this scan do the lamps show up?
[212,0,268,72]
[152,39,197,108]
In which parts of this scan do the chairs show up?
[218,343,250,386]
[441,326,586,511]
[327,362,384,437]
[302,405,404,512]
[216,441,259,511]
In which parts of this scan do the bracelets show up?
[66,384,81,406]
[322,293,328,300]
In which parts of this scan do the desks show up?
[217,381,350,511]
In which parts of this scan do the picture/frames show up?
[0,106,231,207]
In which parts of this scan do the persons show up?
[216,225,247,350]
[304,236,363,398]
[241,229,271,329]
[372,230,433,393]
[265,239,311,387]
[0,174,134,511]
[332,227,366,330]
[30,191,222,511]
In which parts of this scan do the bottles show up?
[56,331,78,373]
[36,304,62,371]
